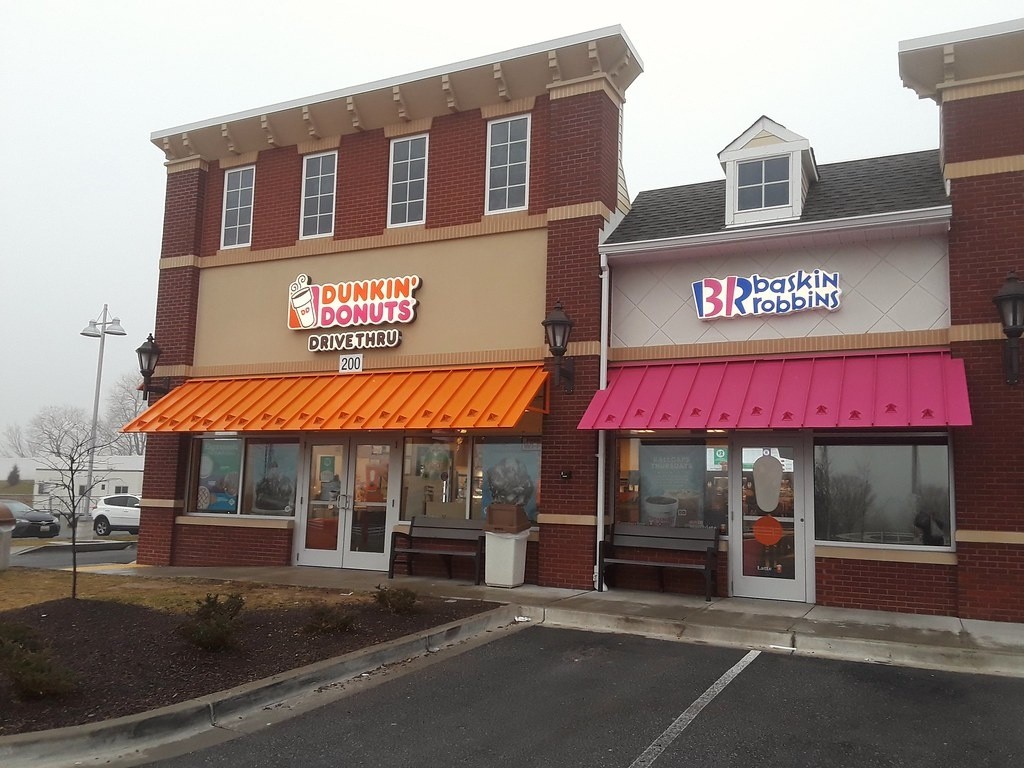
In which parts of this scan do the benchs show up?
[598,522,721,602]
[389,516,487,585]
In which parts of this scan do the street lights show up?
[80,316,127,522]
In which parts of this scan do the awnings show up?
[577,352,972,430]
[118,362,552,433]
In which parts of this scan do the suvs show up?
[91,493,140,536]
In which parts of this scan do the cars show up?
[1,499,60,540]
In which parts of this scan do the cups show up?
[644,496,679,527]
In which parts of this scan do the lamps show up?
[541,298,576,394]
[993,269,1024,385]
[135,333,169,401]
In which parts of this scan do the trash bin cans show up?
[484,503,532,588]
[0,501,17,572]
